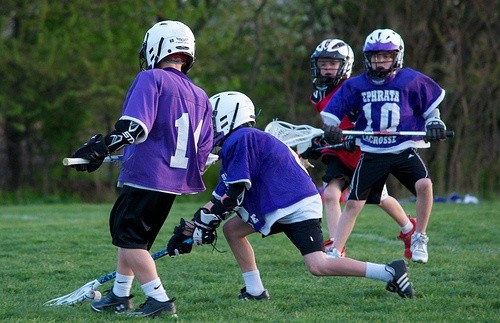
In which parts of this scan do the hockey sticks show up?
[264,120,456,148]
[295,143,344,168]
[62,151,219,174]
[42,237,193,306]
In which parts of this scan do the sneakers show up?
[127,295,177,319]
[386,259,412,299]
[238,287,270,301]
[90,286,133,315]
[410,233,429,263]
[399,216,418,259]
[324,240,346,257]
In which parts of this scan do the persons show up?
[308,38,417,259]
[320,30,448,265]
[73,19,214,319]
[168,90,415,302]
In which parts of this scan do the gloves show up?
[70,133,112,173]
[167,219,196,257]
[192,207,223,246]
[424,121,446,144]
[324,125,343,145]
[345,134,356,152]
[300,137,322,160]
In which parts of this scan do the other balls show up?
[92,290,101,302]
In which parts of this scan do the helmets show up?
[309,39,354,93]
[362,29,404,85]
[208,91,256,137]
[143,20,196,69]
